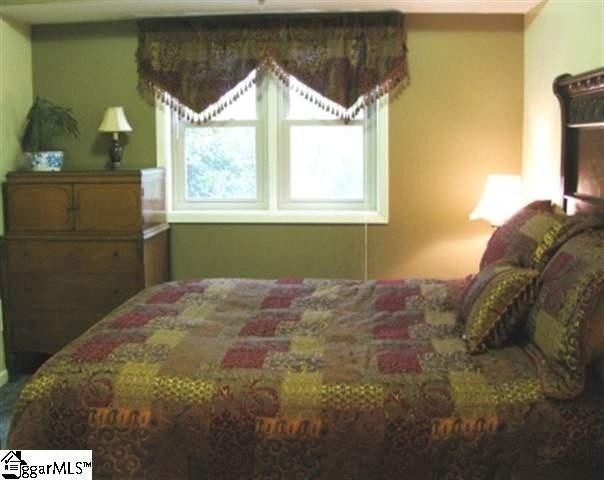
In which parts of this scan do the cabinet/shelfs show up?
[0,167,172,381]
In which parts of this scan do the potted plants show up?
[20,93,82,171]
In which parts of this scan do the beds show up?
[0,66,604,479]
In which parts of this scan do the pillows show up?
[476,192,586,276]
[515,213,603,401]
[456,252,544,358]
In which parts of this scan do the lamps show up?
[95,105,134,168]
[466,173,540,229]
